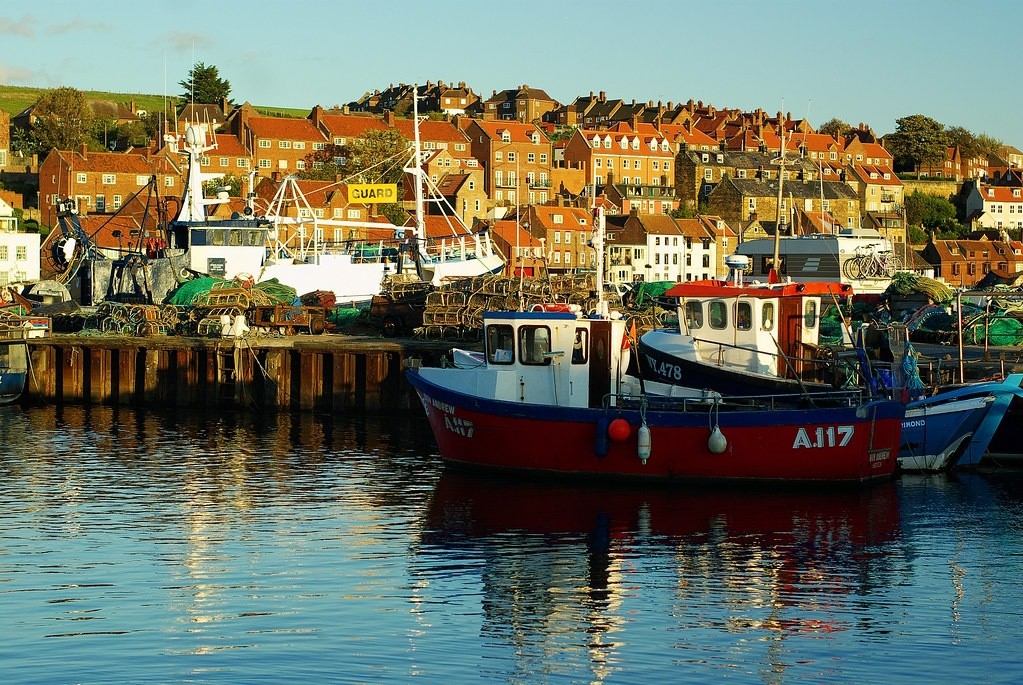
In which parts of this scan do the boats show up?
[43,40,508,306]
[404,207,1023,481]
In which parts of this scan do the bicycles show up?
[850,245,889,278]
[859,243,903,278]
